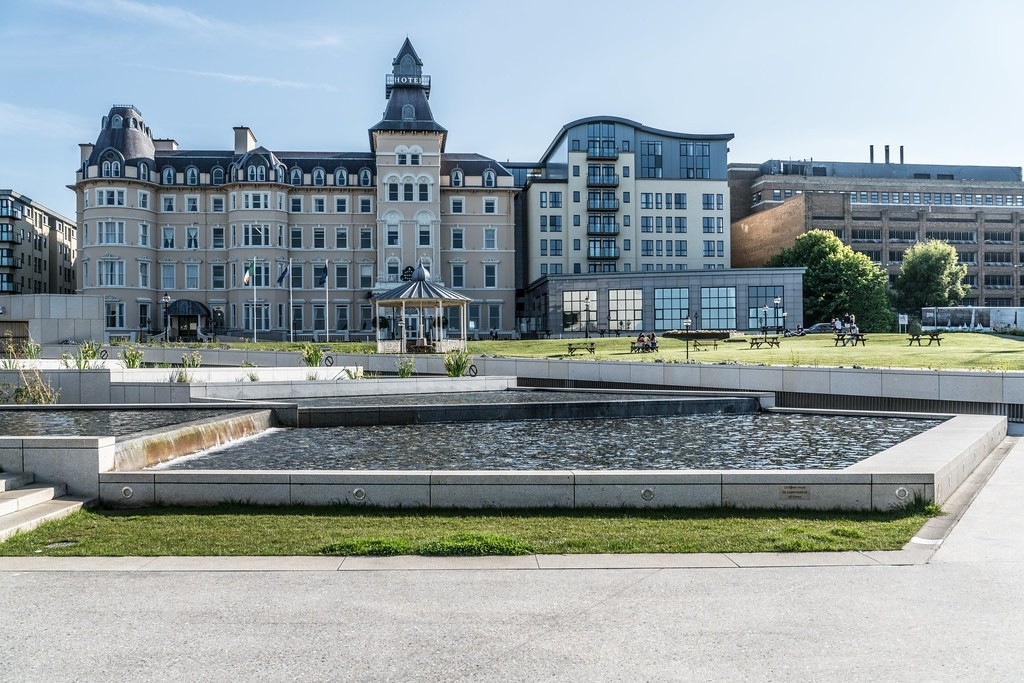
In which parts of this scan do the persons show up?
[830,312,857,333]
[693,339,719,352]
[784,323,806,336]
[636,332,659,352]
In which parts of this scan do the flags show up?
[277,265,289,287]
[319,259,328,287]
[243,261,254,285]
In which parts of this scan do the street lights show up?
[585,297,591,338]
[683,316,692,358]
[774,296,780,334]
[294,320,297,342]
[163,292,170,343]
[762,304,768,342]
[398,319,404,353]
[608,315,611,337]
[695,312,698,330]
[783,313,787,334]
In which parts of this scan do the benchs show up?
[749,337,780,350]
[761,326,784,334]
[530,330,552,339]
[908,333,941,346]
[835,333,866,346]
[693,340,718,351]
[817,325,841,333]
[598,329,620,337]
[630,342,659,353]
[567,343,595,356]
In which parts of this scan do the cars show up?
[800,323,834,333]
